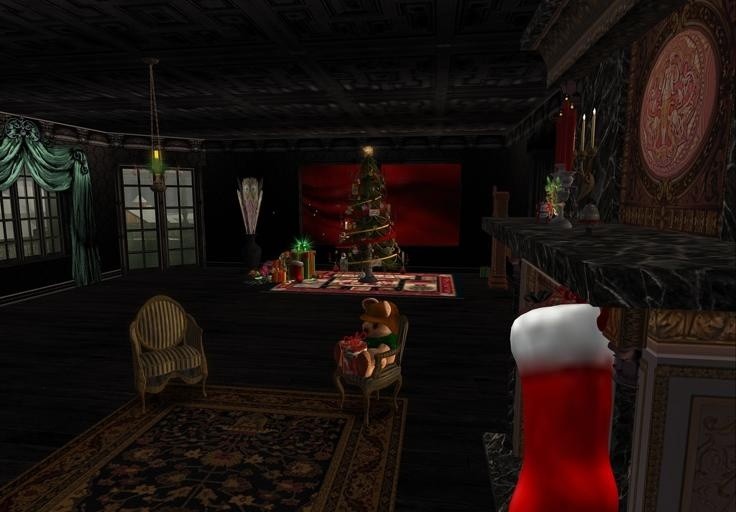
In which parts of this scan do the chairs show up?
[332,314,409,428]
[129,295,209,416]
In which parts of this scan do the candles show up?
[591,107,597,148]
[580,114,586,150]
[143,57,167,192]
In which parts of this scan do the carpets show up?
[270,270,456,297]
[1,382,408,511]
